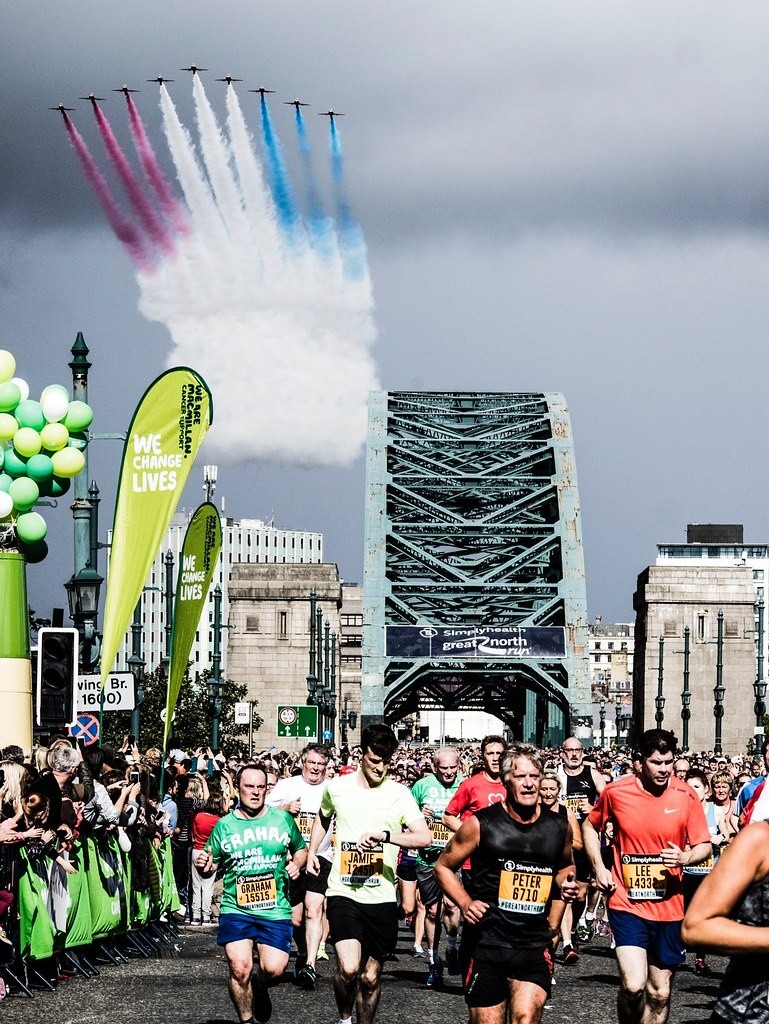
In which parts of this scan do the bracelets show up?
[383,830,391,843]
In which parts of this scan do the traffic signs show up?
[276,704,319,739]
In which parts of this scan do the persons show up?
[195,766,308,1024]
[682,818,769,1024]
[1,738,240,1001]
[433,742,581,1024]
[534,737,607,967]
[442,735,508,993]
[307,722,432,1024]
[266,741,335,990]
[245,735,768,804]
[410,748,469,990]
[581,728,714,1024]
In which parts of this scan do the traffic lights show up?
[36,625,79,730]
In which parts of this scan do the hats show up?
[718,758,727,764]
[214,754,226,763]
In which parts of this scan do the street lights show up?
[597,696,608,749]
[156,549,188,750]
[613,692,623,749]
[62,479,114,673]
[304,586,338,750]
[646,634,667,730]
[671,624,693,753]
[704,608,726,758]
[741,596,768,754]
[123,585,162,753]
[205,582,228,754]
[339,697,358,746]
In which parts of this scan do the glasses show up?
[738,782,745,786]
[564,748,583,752]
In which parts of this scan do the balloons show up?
[1,349,94,567]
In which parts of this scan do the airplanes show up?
[111,83,140,97]
[179,63,210,74]
[146,74,176,85]
[79,93,107,106]
[214,72,243,87]
[318,108,345,120]
[47,103,77,115]
[283,97,311,108]
[247,84,277,95]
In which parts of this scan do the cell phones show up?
[128,734,135,750]
[201,748,208,754]
[156,811,165,821]
[186,774,197,779]
[131,771,139,785]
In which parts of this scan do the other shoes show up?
[550,972,556,985]
[411,945,426,958]
[288,945,298,961]
[219,947,229,962]
[250,975,272,1024]
[184,915,219,927]
[251,948,259,960]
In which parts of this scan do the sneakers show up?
[446,947,460,975]
[694,959,711,975]
[564,945,579,965]
[678,947,686,965]
[427,957,444,987]
[576,919,617,958]
[295,952,307,977]
[298,962,316,990]
[317,948,329,961]
[571,937,579,954]
